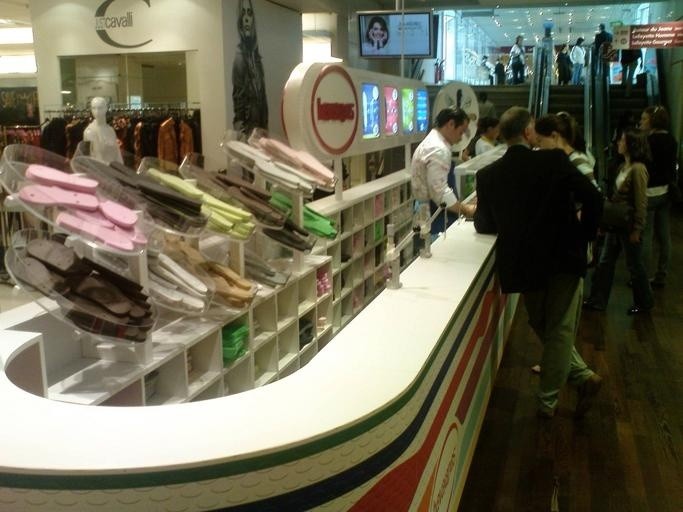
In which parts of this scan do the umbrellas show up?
[582,297,605,312]
[628,305,645,315]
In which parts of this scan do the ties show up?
[354,8,433,59]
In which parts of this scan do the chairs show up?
[626,278,664,291]
[575,373,602,416]
[536,403,560,418]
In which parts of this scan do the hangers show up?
[8,102,200,124]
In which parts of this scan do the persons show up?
[408,104,470,259]
[495,60,506,84]
[594,23,613,50]
[473,104,603,417]
[570,38,586,86]
[529,110,603,374]
[481,56,493,85]
[638,106,680,288]
[362,14,389,55]
[555,43,573,86]
[475,117,500,157]
[509,36,526,85]
[82,97,123,165]
[231,1,268,139]
[620,49,643,99]
[581,130,656,313]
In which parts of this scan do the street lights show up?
[600,201,634,235]
[508,52,526,69]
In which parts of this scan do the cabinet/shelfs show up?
[0,126,521,511]
[380,224,401,290]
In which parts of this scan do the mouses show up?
[8,102,200,124]
